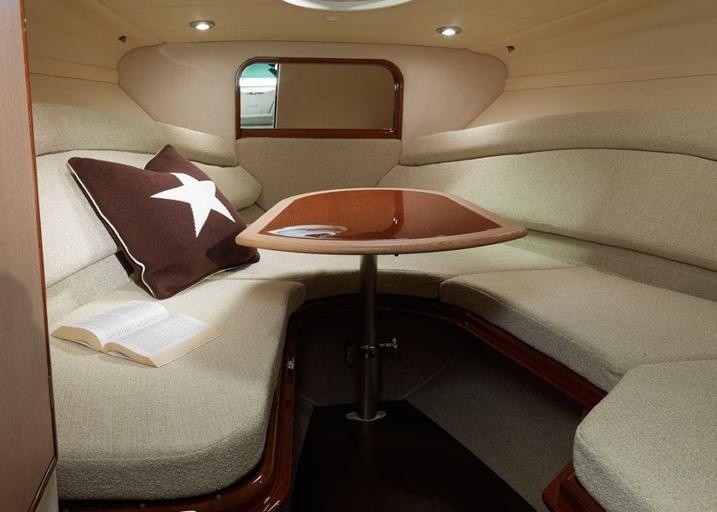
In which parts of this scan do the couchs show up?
[31,101,717,512]
[240,90,276,125]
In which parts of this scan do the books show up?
[49,299,225,368]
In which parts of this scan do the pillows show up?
[66,144,260,299]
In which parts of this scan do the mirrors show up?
[235,55,404,139]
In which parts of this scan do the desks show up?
[235,189,530,424]
